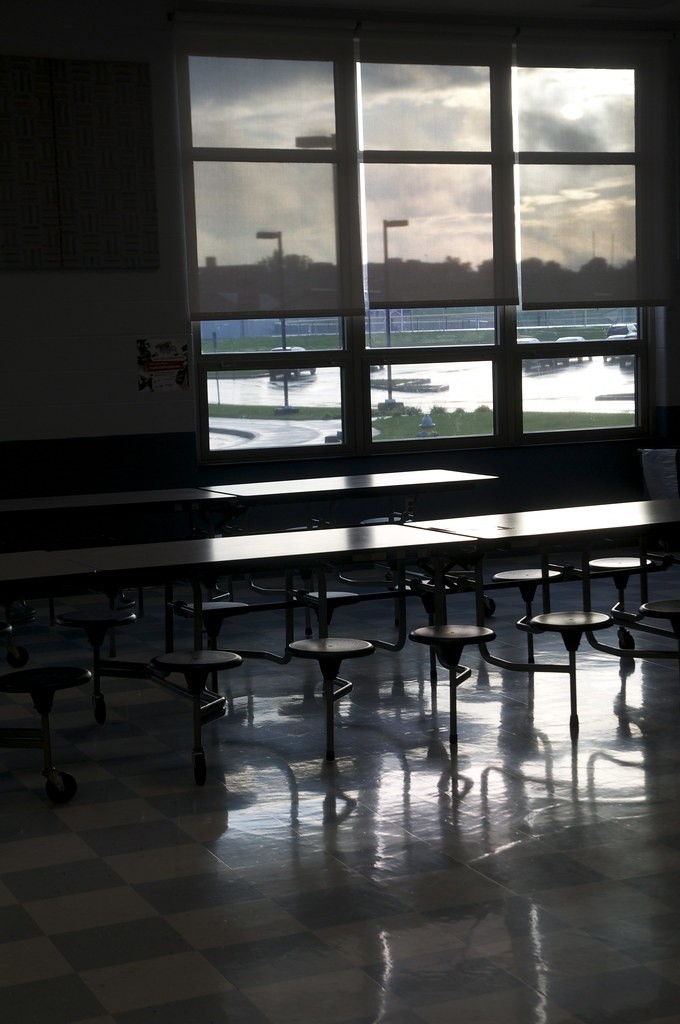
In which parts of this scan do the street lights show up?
[257,230,289,407]
[384,220,409,401]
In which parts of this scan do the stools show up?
[0,513,680,804]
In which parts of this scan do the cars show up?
[604,335,627,363]
[516,338,541,344]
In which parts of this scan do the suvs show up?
[524,342,569,372]
[619,333,636,368]
[605,322,637,339]
[557,337,592,362]
[268,346,316,380]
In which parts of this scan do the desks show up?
[199,469,500,637]
[403,500,680,741]
[49,522,480,698]
[0,489,238,627]
[0,551,99,598]
